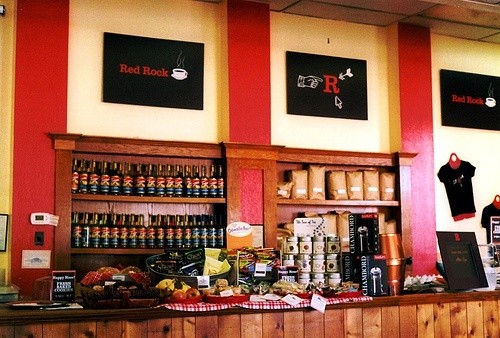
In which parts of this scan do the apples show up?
[171,288,200,300]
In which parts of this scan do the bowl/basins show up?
[205,295,248,305]
[277,291,323,299]
[146,254,232,290]
[166,294,202,306]
[326,290,363,298]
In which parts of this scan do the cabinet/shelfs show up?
[47,132,227,270]
[276,148,420,259]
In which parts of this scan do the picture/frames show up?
[21,250,51,269]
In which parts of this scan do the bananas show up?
[156,278,191,292]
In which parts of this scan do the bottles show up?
[358,227,368,252]
[72,211,227,250]
[71,158,226,198]
[275,235,343,290]
[370,265,381,296]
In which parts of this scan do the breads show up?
[81,265,151,292]
[269,281,307,295]
[203,279,242,296]
